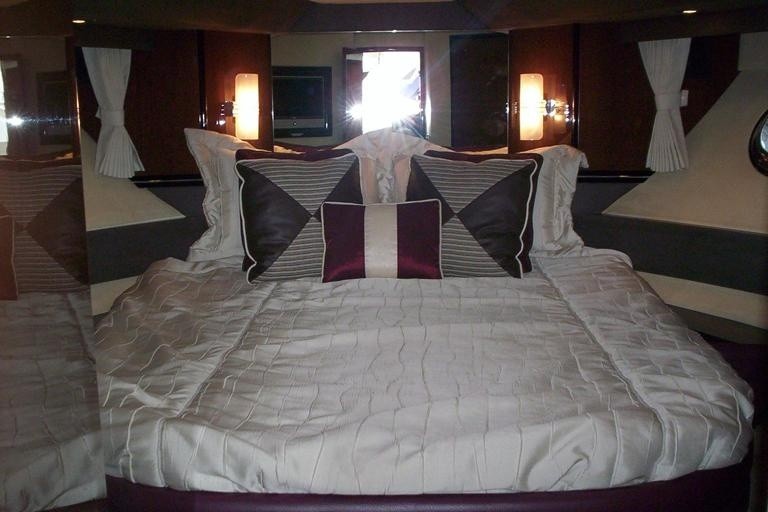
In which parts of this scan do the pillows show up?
[181,126,590,283]
[0,150,90,300]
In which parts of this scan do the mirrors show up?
[0,35,108,512]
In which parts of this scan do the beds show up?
[0,289,108,512]
[95,257,757,512]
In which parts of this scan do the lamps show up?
[216,73,260,140]
[519,73,554,142]
